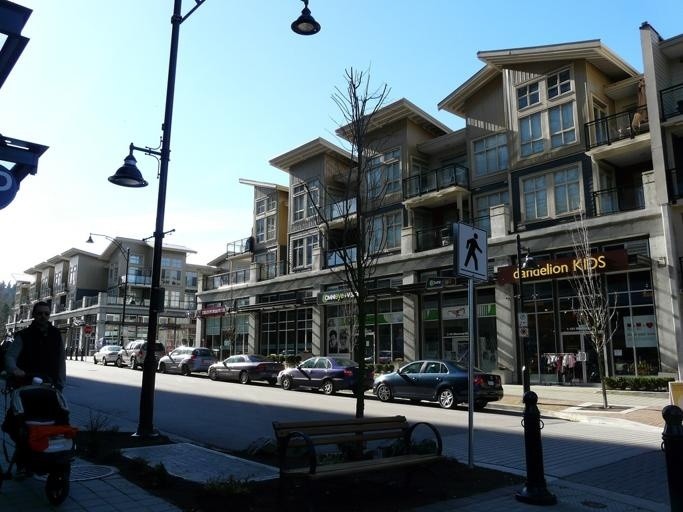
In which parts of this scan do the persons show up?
[339,328,349,353]
[4,301,66,479]
[63,347,85,361]
[329,330,338,353]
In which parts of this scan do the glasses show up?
[36,311,49,315]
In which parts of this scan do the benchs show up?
[268,415,450,509]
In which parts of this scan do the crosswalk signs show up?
[452,221,489,280]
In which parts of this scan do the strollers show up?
[1,374,83,506]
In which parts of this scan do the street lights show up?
[516,234,541,403]
[107,1,321,446]
[86,232,130,337]
